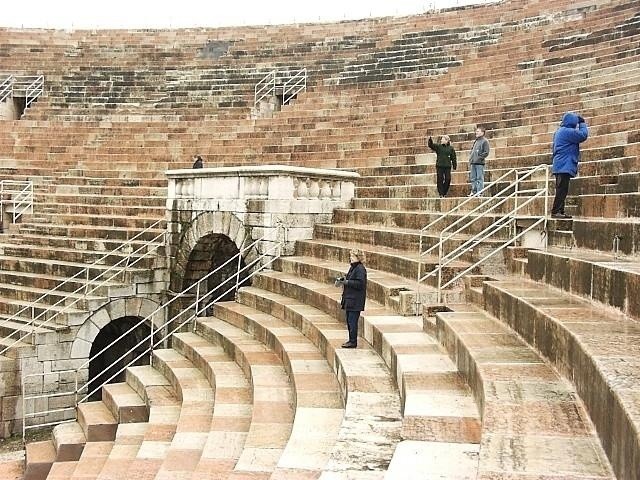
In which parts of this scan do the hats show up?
[350,248,365,260]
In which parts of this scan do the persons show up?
[193,155,203,168]
[340,248,367,348]
[428,135,457,198]
[467,128,489,196]
[551,113,588,219]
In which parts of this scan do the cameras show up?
[337,276,344,281]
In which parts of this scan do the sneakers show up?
[466,192,483,197]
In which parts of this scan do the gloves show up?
[335,276,348,287]
[578,116,585,123]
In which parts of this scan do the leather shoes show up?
[341,341,357,348]
[551,212,572,218]
